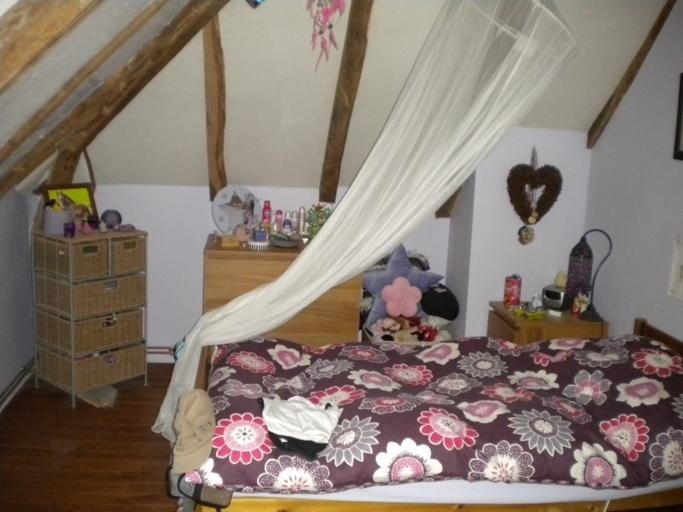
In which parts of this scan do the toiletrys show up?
[263,201,310,252]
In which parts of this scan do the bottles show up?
[260,200,307,236]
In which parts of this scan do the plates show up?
[268,236,303,249]
[512,303,547,321]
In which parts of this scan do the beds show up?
[175,316,682,512]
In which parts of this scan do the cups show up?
[503,277,523,313]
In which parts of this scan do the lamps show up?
[566,228,613,321]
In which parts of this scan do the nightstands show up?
[487,300,609,346]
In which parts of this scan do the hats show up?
[172,390,216,476]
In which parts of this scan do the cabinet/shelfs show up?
[33,226,149,409]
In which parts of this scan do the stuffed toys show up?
[361,242,444,330]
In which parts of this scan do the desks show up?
[202,232,361,349]
